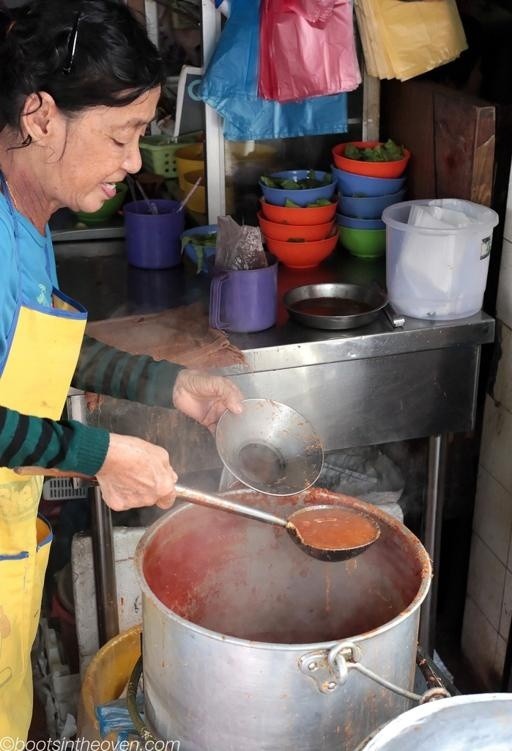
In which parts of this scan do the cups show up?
[205,252,279,335]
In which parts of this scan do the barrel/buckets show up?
[382,197,498,323]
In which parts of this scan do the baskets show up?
[139,134,198,178]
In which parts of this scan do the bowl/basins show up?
[181,224,222,273]
[214,398,325,497]
[257,142,411,268]
[283,279,389,329]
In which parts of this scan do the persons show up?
[0,0,245,751]
